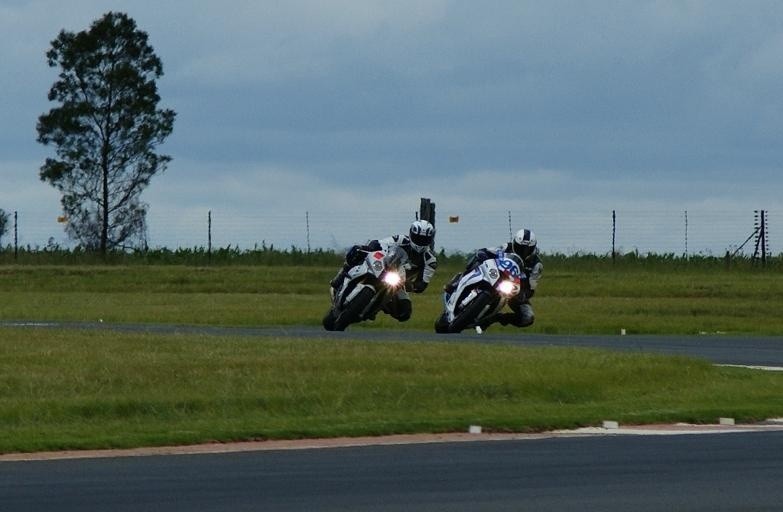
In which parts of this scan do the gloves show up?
[517,291,528,303]
[367,239,382,252]
[405,281,414,292]
[476,250,488,261]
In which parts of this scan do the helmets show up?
[512,228,537,261]
[409,220,435,253]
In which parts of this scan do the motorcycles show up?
[433,249,528,334]
[323,246,421,331]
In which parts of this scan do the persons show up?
[327,219,438,322]
[442,228,544,334]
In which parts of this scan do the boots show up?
[480,311,511,331]
[369,300,392,320]
[443,271,463,294]
[329,261,351,291]
